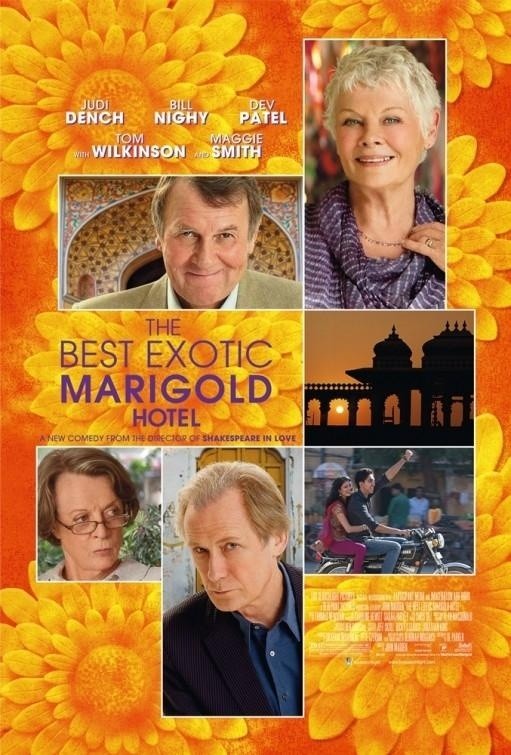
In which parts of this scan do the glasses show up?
[55,504,130,535]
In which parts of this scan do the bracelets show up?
[363,523,366,531]
[402,455,408,462]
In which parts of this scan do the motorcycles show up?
[310,526,473,575]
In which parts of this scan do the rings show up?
[425,238,433,246]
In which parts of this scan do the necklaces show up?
[357,229,406,246]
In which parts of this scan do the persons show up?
[347,448,413,574]
[318,476,369,574]
[38,448,161,581]
[383,483,442,530]
[305,43,445,309]
[71,176,302,309]
[163,461,302,716]
